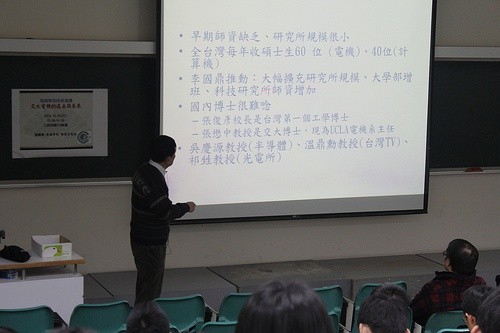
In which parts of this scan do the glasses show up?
[462,314,467,323]
[443,251,447,256]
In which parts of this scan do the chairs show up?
[0,281,470,333]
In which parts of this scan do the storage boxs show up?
[31,234,72,259]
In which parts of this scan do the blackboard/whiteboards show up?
[0,51,500,188]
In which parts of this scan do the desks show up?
[0,252,85,329]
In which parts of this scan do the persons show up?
[126,300,170,333]
[459,282,500,333]
[234,277,334,333]
[409,238,487,333]
[129,135,197,310]
[469,289,500,333]
[357,282,412,333]
